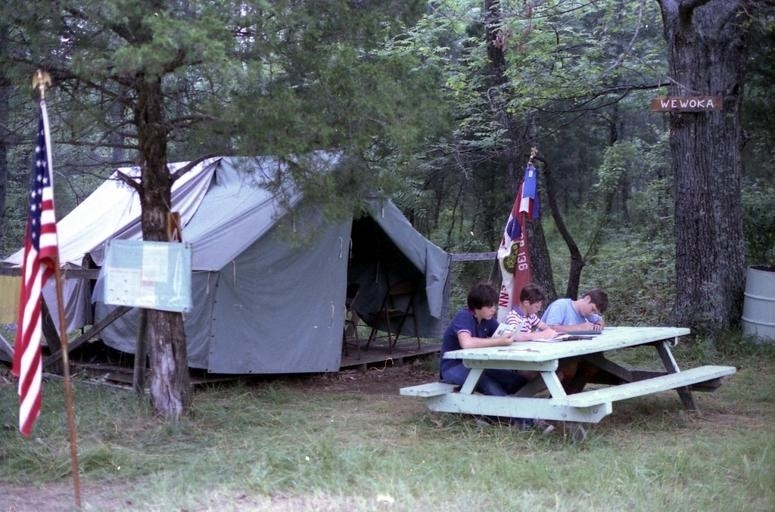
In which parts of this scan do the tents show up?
[0,143,456,394]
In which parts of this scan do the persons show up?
[440,284,556,436]
[503,285,557,341]
[539,289,609,334]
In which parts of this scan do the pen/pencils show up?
[584,318,589,323]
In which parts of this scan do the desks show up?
[443,327,697,424]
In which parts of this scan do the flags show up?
[492,161,542,324]
[12,102,58,436]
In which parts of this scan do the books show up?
[492,322,517,338]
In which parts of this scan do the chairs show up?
[355,261,423,352]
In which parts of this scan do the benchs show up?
[399,350,461,423]
[550,363,739,423]
